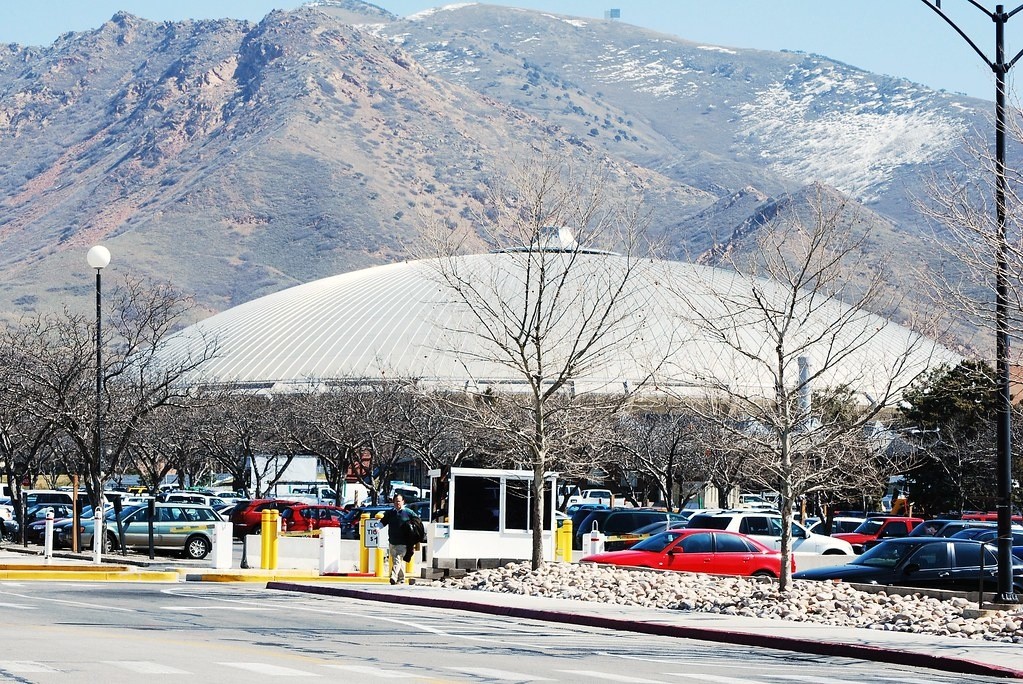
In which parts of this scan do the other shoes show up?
[400,579,405,583]
[390,577,395,585]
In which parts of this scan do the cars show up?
[0,474,1023,595]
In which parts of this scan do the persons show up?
[373,494,418,585]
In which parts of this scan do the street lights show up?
[85,243,113,557]
